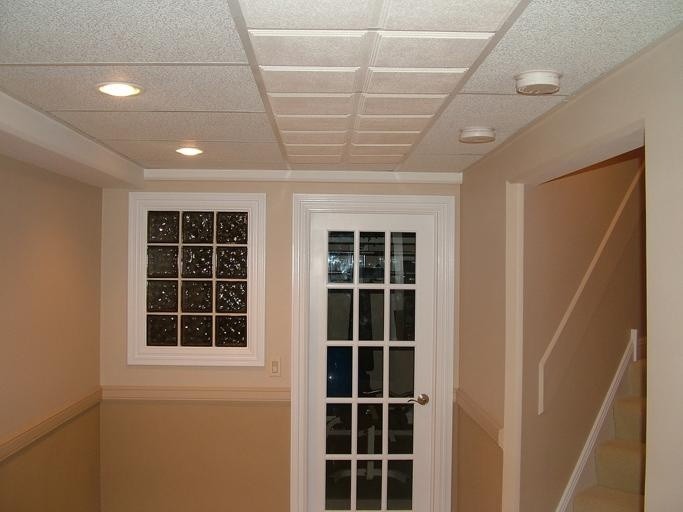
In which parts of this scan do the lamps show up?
[176,147,203,156]
[98,83,145,98]
